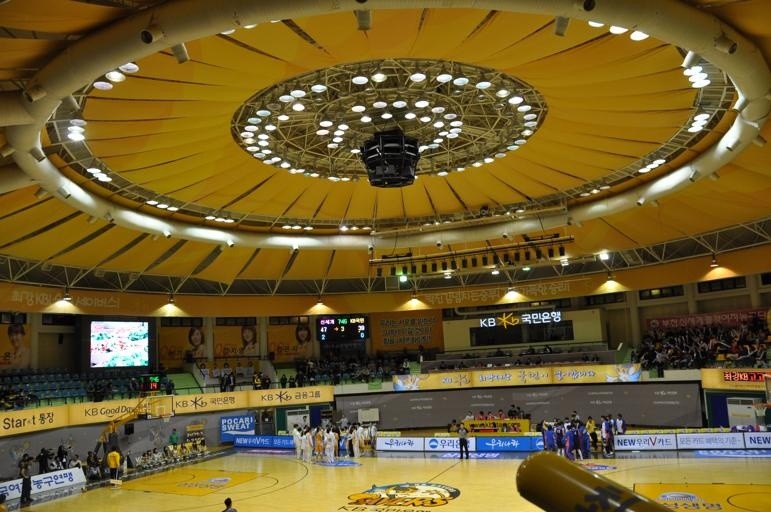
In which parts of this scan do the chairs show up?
[0,371,145,407]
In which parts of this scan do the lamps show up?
[291,243,300,252]
[679,33,738,76]
[634,99,769,216]
[364,218,577,277]
[134,23,194,65]
[358,135,419,188]
[353,9,375,32]
[552,16,573,40]
[2,81,177,251]
[226,241,235,247]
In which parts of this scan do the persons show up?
[629,311,771,379]
[449,411,628,462]
[0,388,39,410]
[279,348,409,388]
[292,414,379,465]
[419,344,425,359]
[129,376,174,399]
[0,324,31,370]
[240,324,260,355]
[188,326,205,358]
[201,362,271,392]
[222,497,238,511]
[0,494,8,511]
[440,345,553,370]
[87,381,113,402]
[582,353,599,362]
[294,324,312,359]
[16,427,209,503]
